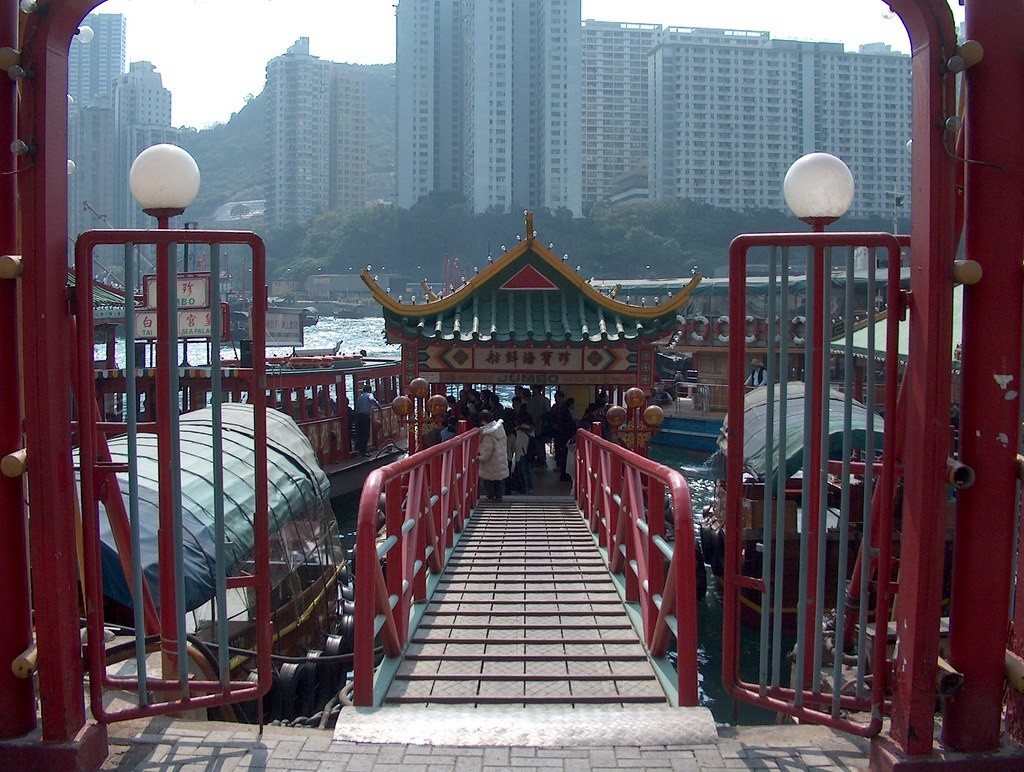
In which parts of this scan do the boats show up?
[61,214,965,715]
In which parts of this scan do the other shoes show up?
[494,497,502,503]
[561,473,571,481]
[554,465,562,472]
[359,451,372,457]
[485,499,494,503]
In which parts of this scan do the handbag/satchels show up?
[541,399,552,436]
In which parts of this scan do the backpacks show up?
[517,428,538,464]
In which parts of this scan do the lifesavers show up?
[790,316,806,345]
[763,314,780,341]
[676,313,688,339]
[744,315,759,343]
[715,315,730,343]
[691,314,710,342]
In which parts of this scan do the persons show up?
[356,385,381,458]
[744,359,768,389]
[305,391,355,452]
[423,383,612,502]
